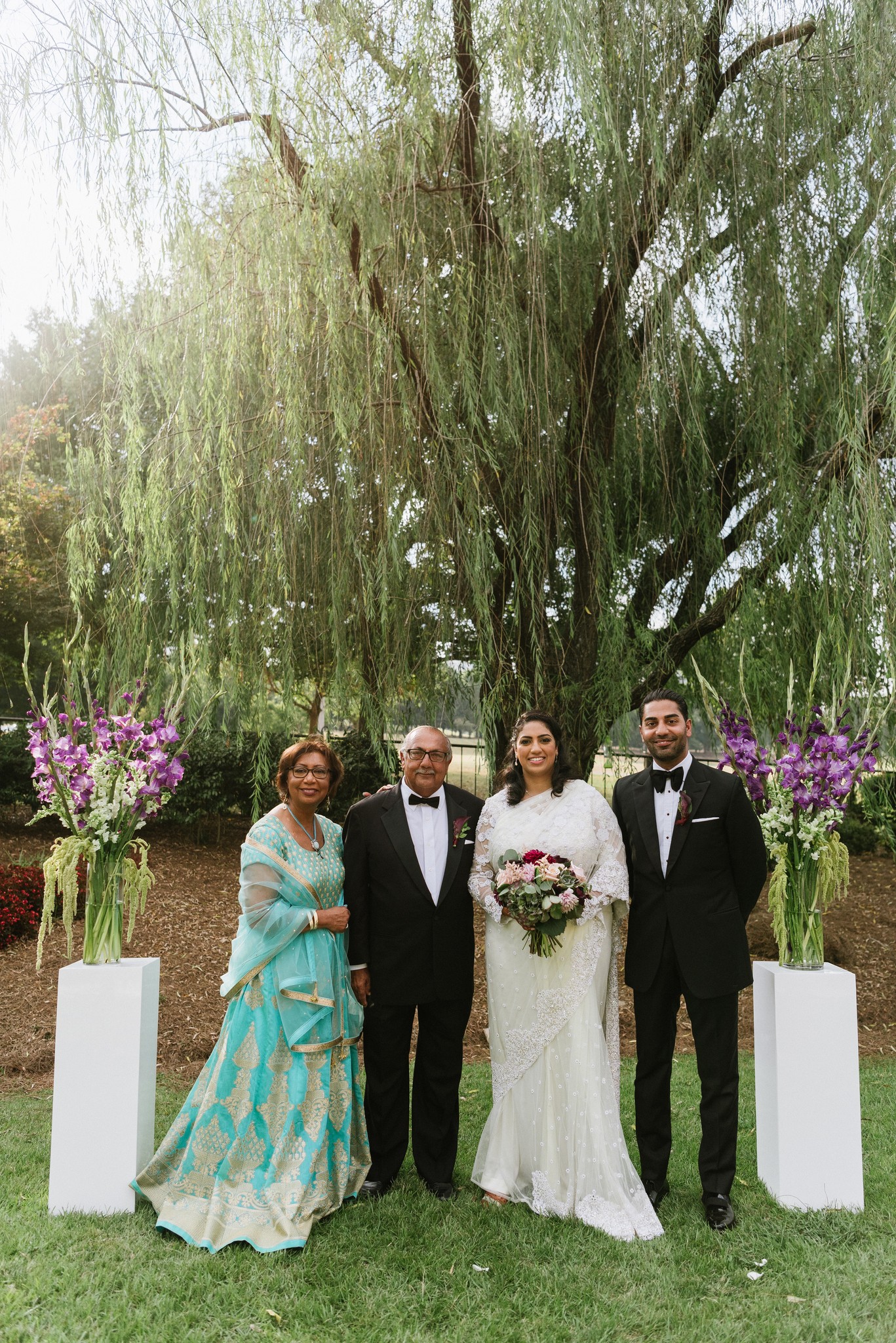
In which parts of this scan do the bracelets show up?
[306,910,314,931]
[310,909,318,931]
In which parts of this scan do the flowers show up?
[489,848,593,959]
[20,621,226,975]
[688,632,896,972]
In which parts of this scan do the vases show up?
[81,833,131,966]
[784,869,825,972]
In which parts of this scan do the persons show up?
[608,688,772,1233]
[341,725,489,1209]
[466,708,630,1228]
[208,738,396,1219]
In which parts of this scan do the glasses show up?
[406,749,447,763]
[289,766,331,780]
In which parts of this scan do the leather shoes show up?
[425,1179,455,1200]
[644,1184,668,1208]
[703,1191,737,1230]
[360,1179,394,1197]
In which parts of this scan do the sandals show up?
[480,1192,509,1209]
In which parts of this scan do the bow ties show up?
[648,766,684,793]
[408,793,440,808]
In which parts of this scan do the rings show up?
[345,923,349,929]
[518,921,521,924]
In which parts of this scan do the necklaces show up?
[285,801,325,859]
[525,788,534,800]
